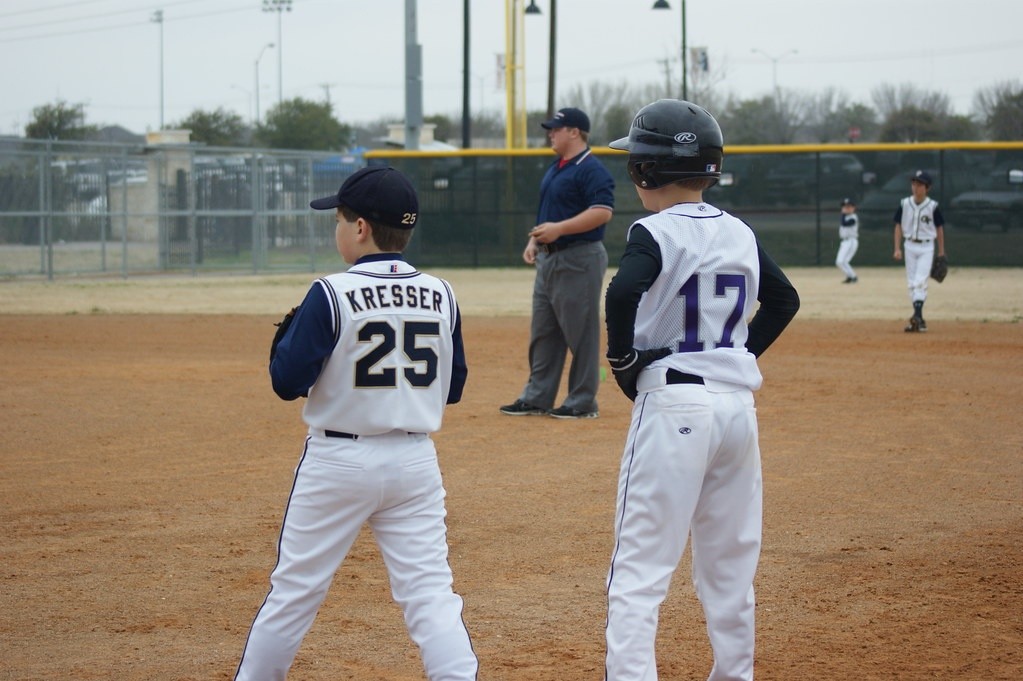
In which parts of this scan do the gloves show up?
[606,347,672,402]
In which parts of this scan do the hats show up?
[840,198,853,206]
[911,170,932,187]
[309,167,419,230]
[541,108,590,133]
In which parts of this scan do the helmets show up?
[608,100,724,190]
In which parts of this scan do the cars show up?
[51,152,313,216]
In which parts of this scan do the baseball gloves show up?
[929,256,948,283]
[270,305,308,397]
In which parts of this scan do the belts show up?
[542,240,587,254]
[666,367,705,384]
[324,430,414,440]
[908,238,929,243]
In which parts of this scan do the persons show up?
[229,166,482,681]
[602,99,801,681]
[834,197,860,283]
[498,105,614,422]
[893,169,945,330]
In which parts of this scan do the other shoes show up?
[910,315,922,323]
[905,323,926,331]
[842,277,857,284]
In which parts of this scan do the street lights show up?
[651,0,689,101]
[262,0,292,106]
[524,0,557,148]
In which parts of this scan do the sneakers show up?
[550,404,600,419]
[500,398,549,415]
